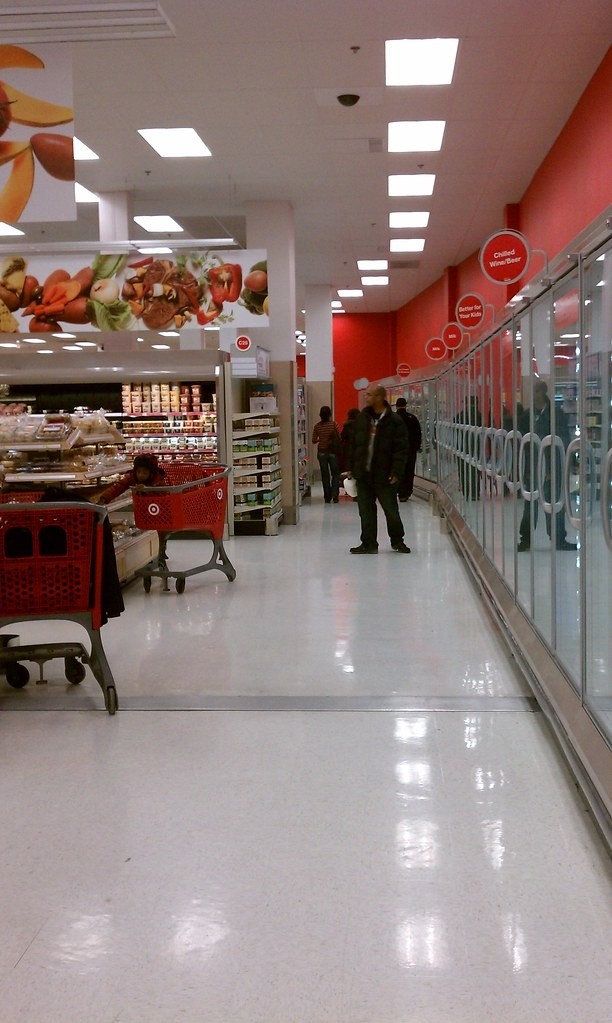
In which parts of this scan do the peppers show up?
[207,254,242,306]
[197,283,223,324]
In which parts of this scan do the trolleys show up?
[0,488,122,716]
[128,461,236,592]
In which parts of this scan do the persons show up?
[453,381,579,552]
[312,406,341,503]
[395,398,421,502]
[344,383,411,554]
[96,453,169,558]
[341,408,361,501]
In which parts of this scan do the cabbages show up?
[88,254,131,332]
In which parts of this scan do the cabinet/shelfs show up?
[230,357,284,537]
[0,414,161,586]
[0,363,229,538]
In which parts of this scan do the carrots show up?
[22,267,95,336]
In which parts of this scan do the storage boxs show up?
[231,383,284,520]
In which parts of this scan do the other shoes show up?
[350,543,377,554]
[324,496,339,503]
[514,540,531,552]
[399,496,409,502]
[551,538,577,551]
[398,543,410,553]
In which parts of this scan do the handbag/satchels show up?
[330,421,343,453]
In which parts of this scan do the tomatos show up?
[30,134,74,182]
[243,270,267,292]
[0,87,17,137]
